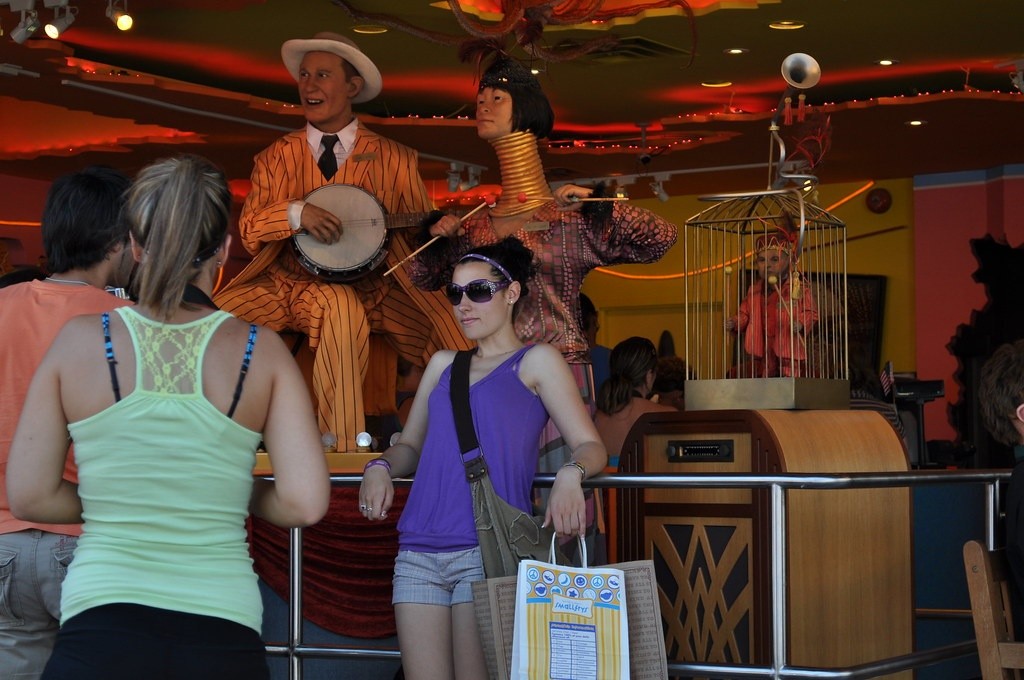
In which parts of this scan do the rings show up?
[367,507,372,511]
[361,504,367,510]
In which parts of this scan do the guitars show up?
[292,182,479,279]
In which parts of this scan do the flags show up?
[881,364,894,396]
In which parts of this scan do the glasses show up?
[446,279,510,305]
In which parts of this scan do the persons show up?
[849,366,907,440]
[1,166,133,679]
[979,339,1024,446]
[726,234,818,377]
[416,56,677,532]
[213,32,476,454]
[358,238,607,679]
[7,161,331,680]
[575,294,677,459]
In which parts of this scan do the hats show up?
[283,32,383,104]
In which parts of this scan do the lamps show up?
[105,0,133,31]
[8,0,39,44]
[44,0,76,39]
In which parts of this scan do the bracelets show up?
[562,462,585,481]
[364,458,391,472]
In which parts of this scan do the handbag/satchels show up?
[509,531,630,680]
[472,476,595,579]
[470,532,669,680]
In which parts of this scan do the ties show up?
[318,135,339,182]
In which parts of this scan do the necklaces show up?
[46,278,87,285]
